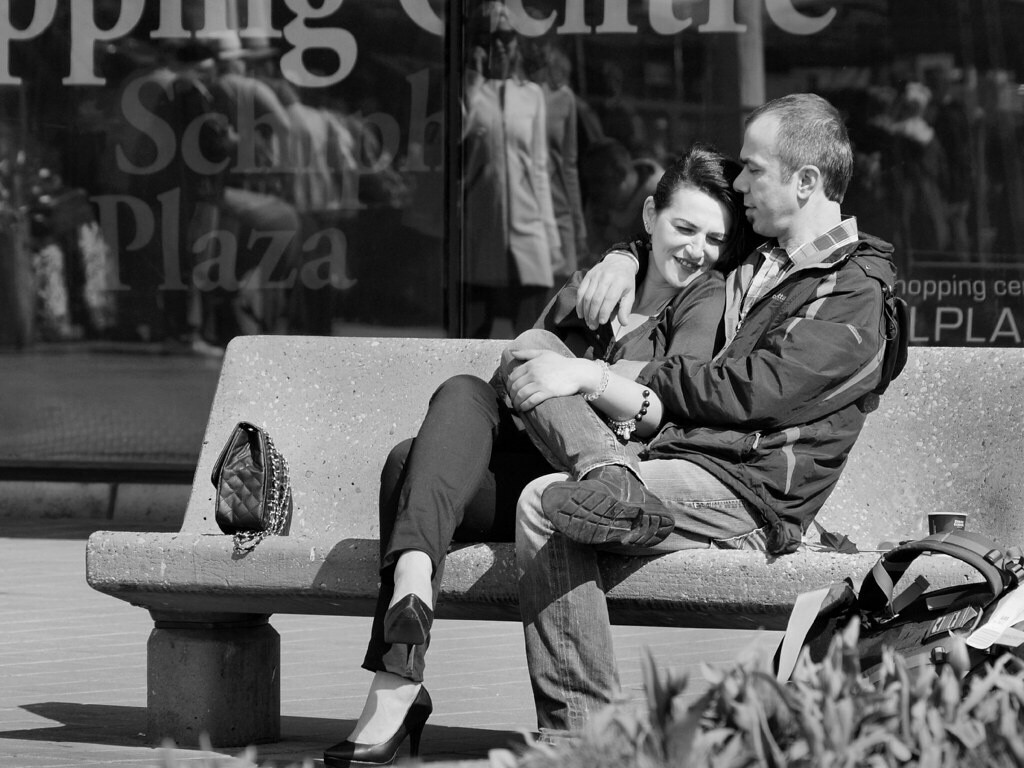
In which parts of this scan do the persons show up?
[323,141,755,768]
[500,93,909,745]
[460,0,591,341]
[98,27,356,350]
[874,82,950,253]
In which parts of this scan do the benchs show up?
[87,333,1024,752]
[0,348,234,521]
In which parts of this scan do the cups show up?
[928,512,968,536]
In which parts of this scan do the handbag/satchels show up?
[211,421,295,553]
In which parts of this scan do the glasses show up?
[471,29,519,49]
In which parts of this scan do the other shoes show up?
[522,731,580,760]
[540,465,676,550]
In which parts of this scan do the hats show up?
[239,30,282,63]
[195,31,250,71]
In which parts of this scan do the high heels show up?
[323,683,434,768]
[382,593,434,677]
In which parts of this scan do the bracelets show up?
[613,250,639,275]
[582,359,610,401]
[607,389,650,440]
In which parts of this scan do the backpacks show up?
[775,529,1024,697]
